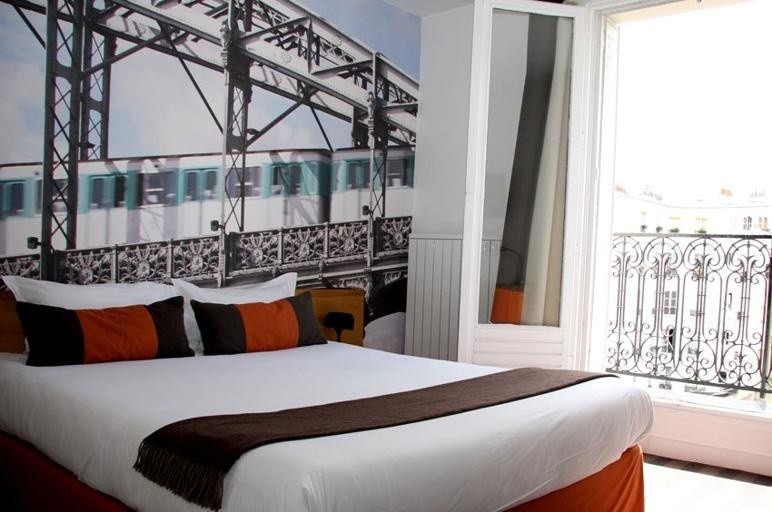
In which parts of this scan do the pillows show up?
[14,294,196,366]
[171,270,298,352]
[1,275,199,354]
[190,290,328,357]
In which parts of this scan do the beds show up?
[0,289,644,512]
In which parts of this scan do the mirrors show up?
[456,2,590,369]
